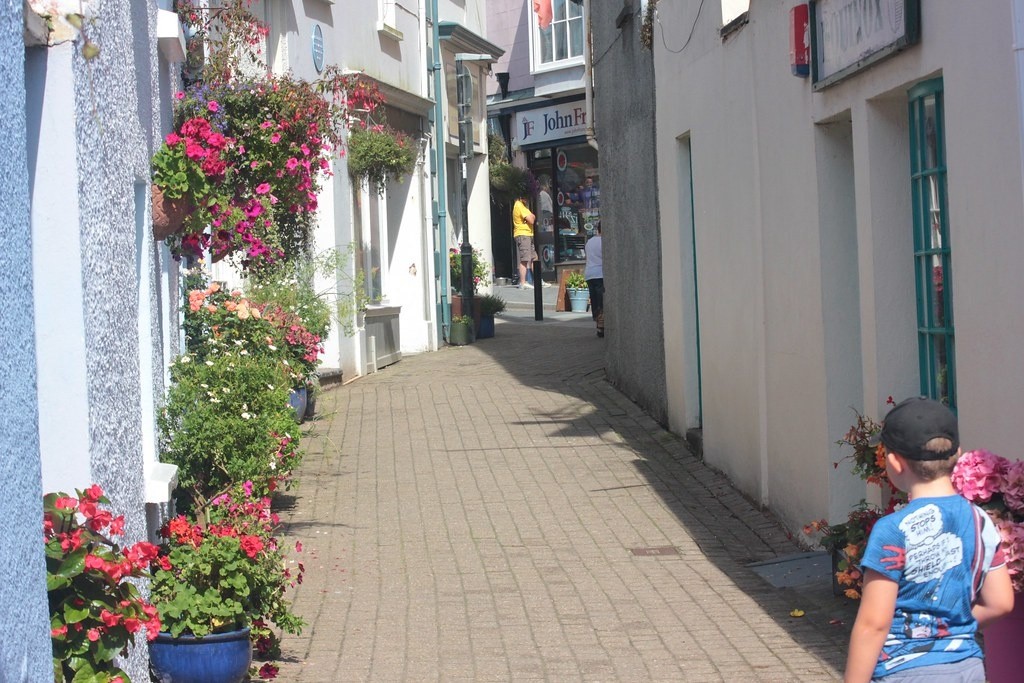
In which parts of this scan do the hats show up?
[868,395,959,461]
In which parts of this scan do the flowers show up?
[35,264,329,683]
[144,2,420,262]
[952,445,1024,597]
[449,246,485,292]
[453,313,474,326]
[802,394,949,601]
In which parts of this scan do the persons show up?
[512,192,552,289]
[539,184,554,253]
[585,221,604,337]
[565,177,599,208]
[845,396,1014,683]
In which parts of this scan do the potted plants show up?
[565,273,592,313]
[479,296,505,337]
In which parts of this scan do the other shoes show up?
[541,279,551,288]
[596,327,604,337]
[519,281,534,290]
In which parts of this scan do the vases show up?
[451,296,481,335]
[287,386,309,422]
[147,624,253,683]
[832,541,871,602]
[450,324,476,346]
[980,591,1024,683]
[151,181,187,241]
